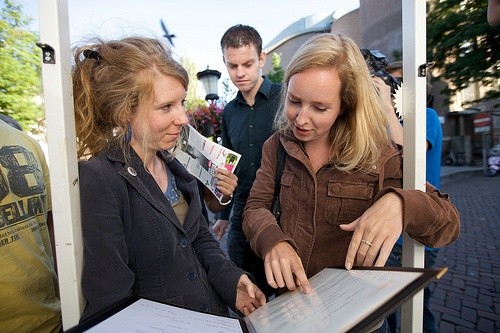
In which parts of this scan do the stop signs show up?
[473,113,491,127]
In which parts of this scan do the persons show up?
[212,25,283,300]
[385,81,444,333]
[242,33,460,333]
[74,37,266,325]
[0,119,64,333]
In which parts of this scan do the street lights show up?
[196,65,222,144]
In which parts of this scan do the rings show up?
[361,240,371,246]
